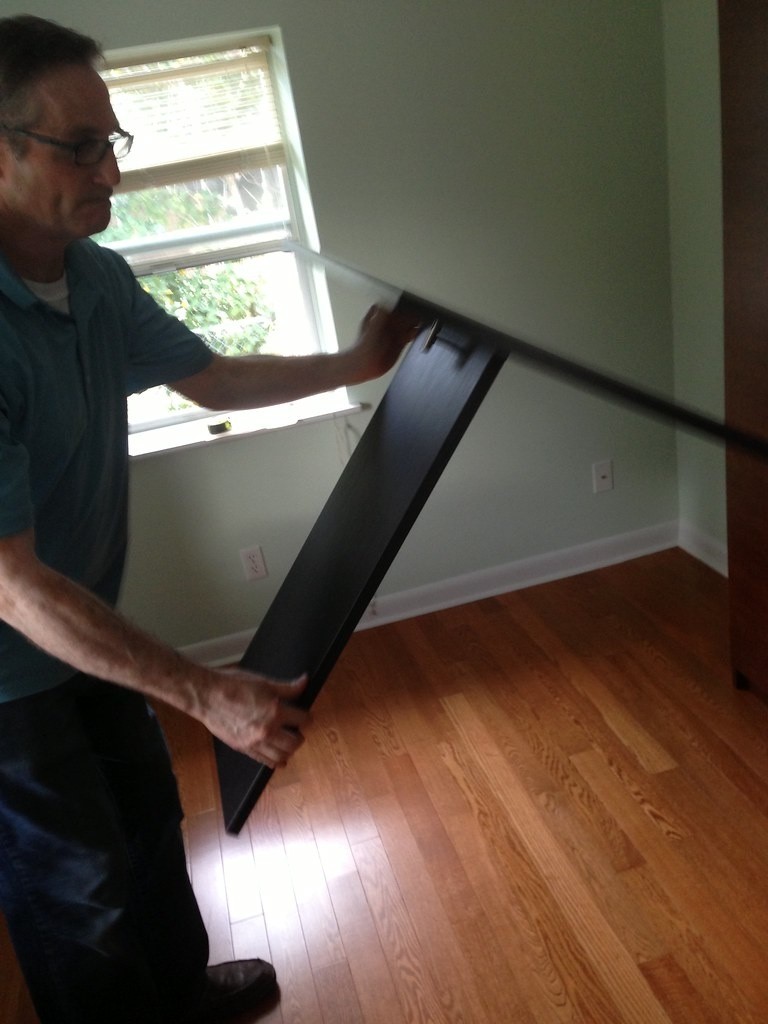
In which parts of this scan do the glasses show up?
[3,124,133,165]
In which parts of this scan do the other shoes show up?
[189,957,278,1019]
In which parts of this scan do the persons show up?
[0,10,419,1020]
